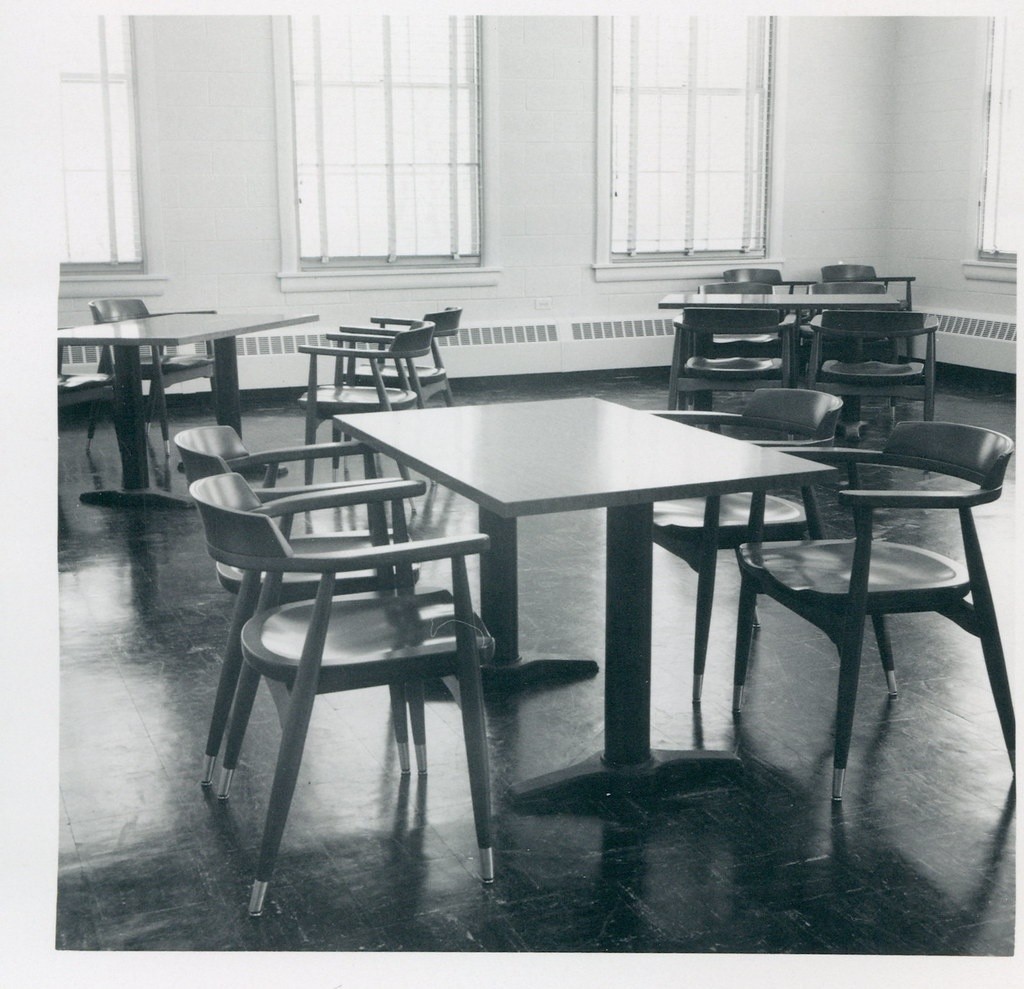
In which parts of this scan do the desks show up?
[57,314,319,510]
[660,293,900,421]
[333,397,840,808]
[782,274,916,311]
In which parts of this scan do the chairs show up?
[733,422,1015,800]
[58,297,216,458]
[669,265,939,444]
[645,388,845,703]
[188,471,496,916]
[174,426,403,789]
[298,308,464,514]
